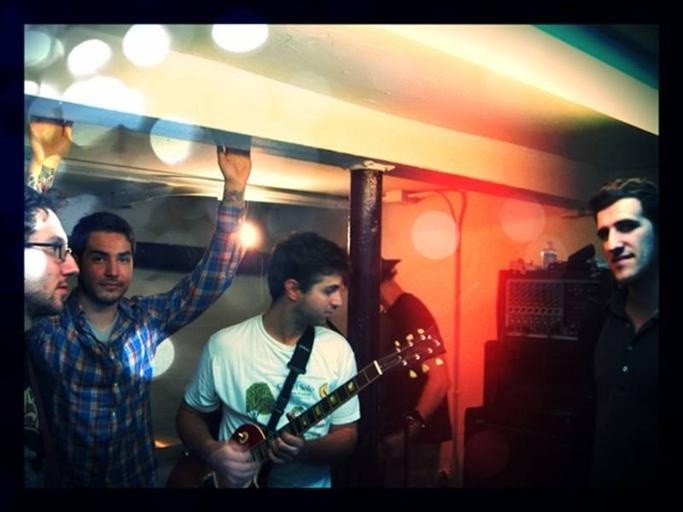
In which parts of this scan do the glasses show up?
[24,239,71,261]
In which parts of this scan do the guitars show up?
[166,328,446,489]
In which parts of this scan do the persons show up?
[176,229,365,488]
[25,115,254,486]
[376,256,454,488]
[548,176,659,489]
[23,188,91,491]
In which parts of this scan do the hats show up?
[383,253,401,281]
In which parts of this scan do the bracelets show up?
[404,411,429,436]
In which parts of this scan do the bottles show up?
[540,240,558,270]
